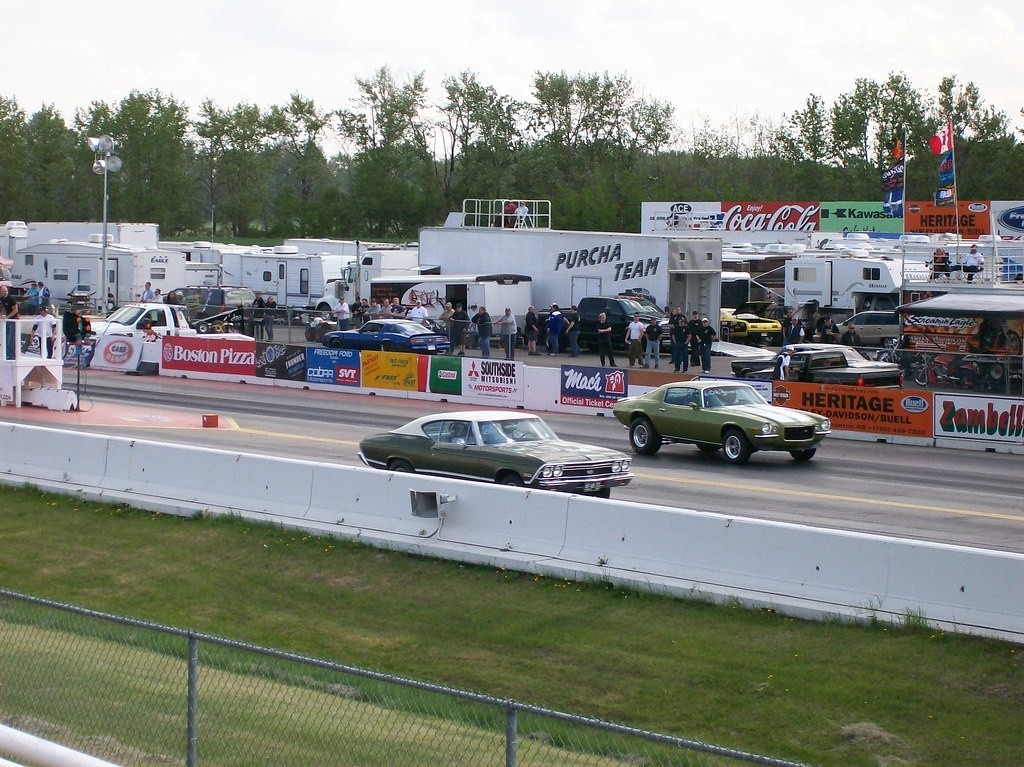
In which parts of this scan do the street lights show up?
[87,134,122,311]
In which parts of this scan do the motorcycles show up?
[875,335,1024,394]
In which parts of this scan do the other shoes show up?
[655,365,659,369]
[549,352,558,356]
[458,351,465,357]
[639,365,643,368]
[528,352,533,355]
[610,363,617,367]
[644,364,649,369]
[669,361,711,374]
[505,358,509,360]
[534,352,541,356]
[511,358,515,361]
[629,366,635,368]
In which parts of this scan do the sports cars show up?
[322,319,450,354]
[357,409,635,500]
[611,380,832,464]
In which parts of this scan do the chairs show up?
[925,252,989,283]
[494,209,532,228]
[449,422,474,445]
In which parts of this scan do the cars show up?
[812,311,902,348]
[720,300,783,338]
[730,344,846,380]
[744,347,904,390]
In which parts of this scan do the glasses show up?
[680,321,684,322]
[634,316,639,317]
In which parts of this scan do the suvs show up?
[163,284,257,320]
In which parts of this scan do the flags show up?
[934,185,955,207]
[928,122,952,155]
[881,159,904,191]
[883,189,903,217]
[938,151,954,187]
[887,131,905,158]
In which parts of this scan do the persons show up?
[945,244,984,284]
[0,284,18,360]
[252,293,277,342]
[773,345,794,381]
[30,307,57,357]
[22,282,50,314]
[782,309,801,345]
[141,282,188,326]
[495,201,529,227]
[624,307,717,373]
[333,297,581,360]
[597,312,618,368]
[820,316,840,344]
[100,287,115,310]
[787,316,805,344]
[842,322,862,345]
[792,365,802,371]
[925,247,950,282]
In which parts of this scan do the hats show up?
[416,298,421,303]
[456,303,462,308]
[786,345,794,352]
[469,304,477,308]
[505,308,511,311]
[702,317,708,322]
[692,311,698,314]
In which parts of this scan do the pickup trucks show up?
[83,303,256,344]
[538,295,691,354]
